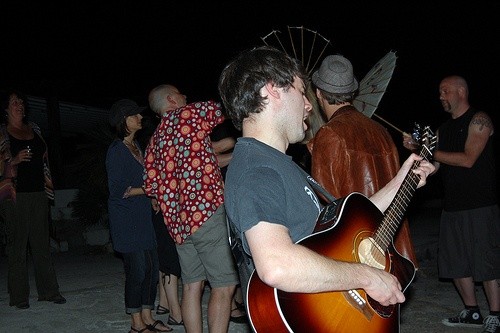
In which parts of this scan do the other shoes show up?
[167,316,184,325]
[229,309,249,324]
[38,295,66,304]
[156,304,170,314]
[234,300,246,312]
[14,301,30,309]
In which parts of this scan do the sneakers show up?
[442,310,483,328]
[481,316,500,333]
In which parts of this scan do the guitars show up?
[246,123,437,333]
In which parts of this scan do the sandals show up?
[145,320,173,332]
[128,326,147,333]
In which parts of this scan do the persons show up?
[311,55,418,269]
[219,46,406,307]
[105,100,173,333]
[0,89,67,309]
[142,85,246,333]
[402,76,500,333]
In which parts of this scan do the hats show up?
[312,55,358,93]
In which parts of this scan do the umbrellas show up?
[352,49,410,140]
